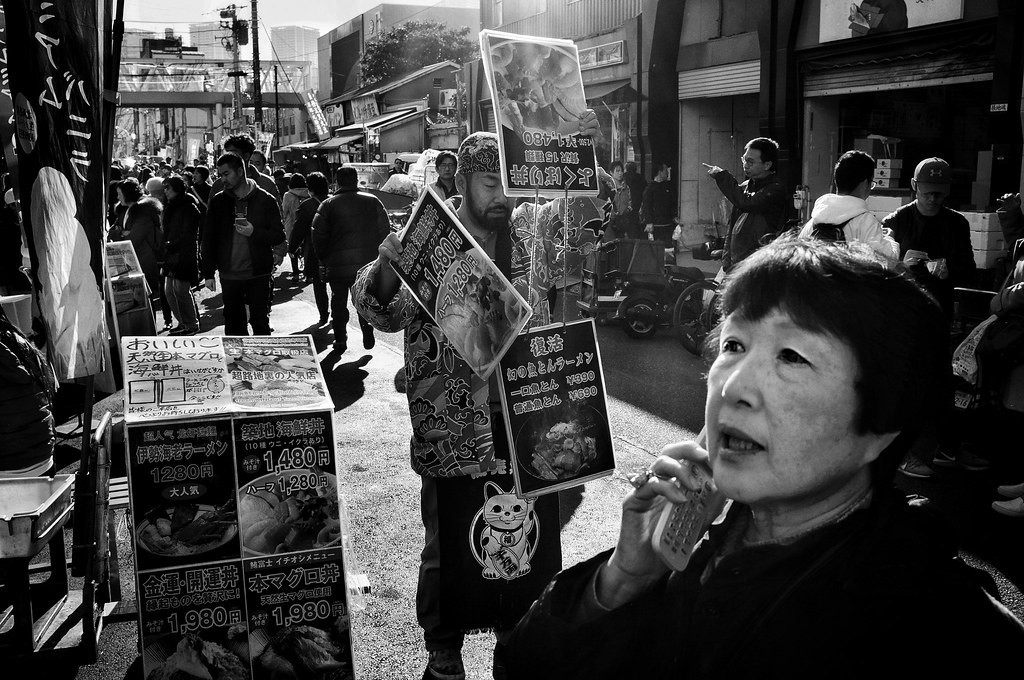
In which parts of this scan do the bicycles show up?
[672,248,728,360]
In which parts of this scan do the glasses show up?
[868,178,877,190]
[741,156,766,166]
[440,164,454,169]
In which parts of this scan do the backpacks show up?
[809,217,854,249]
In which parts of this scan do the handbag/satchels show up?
[976,309,1024,368]
[951,314,998,386]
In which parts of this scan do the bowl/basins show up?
[434,248,522,372]
[136,504,238,558]
[491,40,587,140]
[238,469,341,555]
[924,258,947,275]
[516,402,609,483]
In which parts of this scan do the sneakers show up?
[933,434,989,471]
[992,496,1024,517]
[896,449,937,479]
[169,324,201,336]
[997,483,1024,500]
[427,646,465,680]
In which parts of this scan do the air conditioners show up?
[439,89,458,108]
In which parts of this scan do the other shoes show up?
[362,324,375,349]
[292,278,299,284]
[334,340,345,349]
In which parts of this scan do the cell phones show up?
[235,218,247,226]
[651,426,713,571]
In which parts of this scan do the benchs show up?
[71,477,129,575]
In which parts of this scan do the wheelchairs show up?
[576,235,693,341]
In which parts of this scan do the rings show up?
[628,468,656,489]
[239,229,241,231]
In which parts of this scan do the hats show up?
[914,157,951,195]
[458,132,502,174]
[145,177,165,191]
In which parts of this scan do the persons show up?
[990,192,1024,516]
[882,158,976,480]
[798,151,901,267]
[607,157,674,248]
[348,109,613,680]
[495,235,1024,680]
[428,149,458,202]
[275,167,391,355]
[107,133,288,337]
[701,136,795,281]
[1,309,59,481]
[388,157,408,177]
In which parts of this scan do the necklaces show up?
[739,489,871,548]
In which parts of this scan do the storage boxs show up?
[865,159,914,222]
[961,212,1005,270]
[601,240,665,276]
